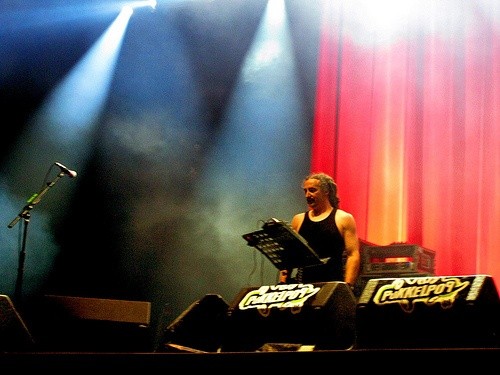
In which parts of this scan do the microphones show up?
[54,162,77,178]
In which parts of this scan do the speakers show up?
[164,294,230,351]
[38,295,155,353]
[355,274,500,347]
[0,294,36,351]
[226,280,358,350]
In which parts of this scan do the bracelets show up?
[347,283,354,291]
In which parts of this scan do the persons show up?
[277,172,361,295]
